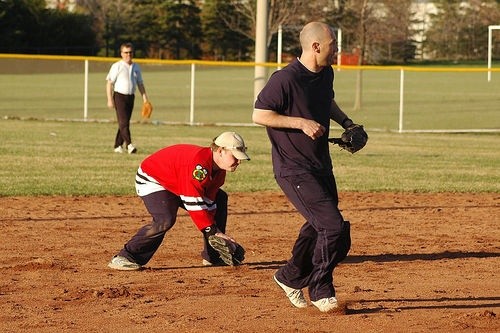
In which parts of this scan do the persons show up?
[105,43,149,155]
[252,22,368,314]
[108,130,250,271]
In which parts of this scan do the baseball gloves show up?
[208,235,246,267]
[141,102,153,118]
[328,125,368,154]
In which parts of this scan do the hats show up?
[214,131,250,161]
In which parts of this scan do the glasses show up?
[123,52,132,54]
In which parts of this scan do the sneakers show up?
[107,254,141,271]
[114,145,124,154]
[272,274,308,310]
[202,258,239,266]
[127,144,136,154]
[310,296,337,313]
